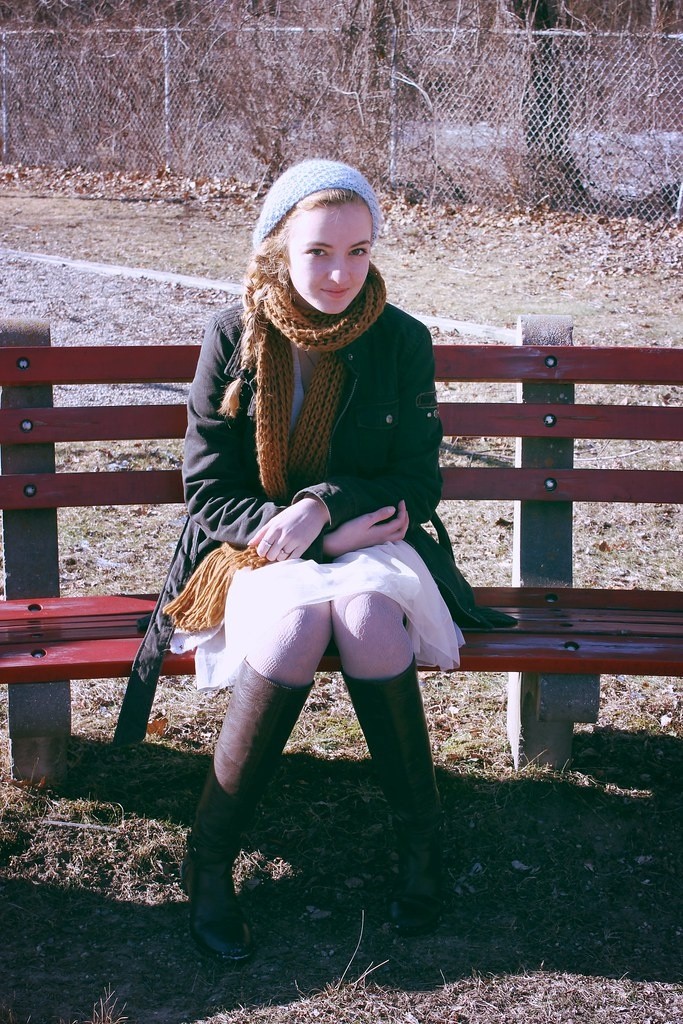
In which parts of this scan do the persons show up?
[111,158,519,959]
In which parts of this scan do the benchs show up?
[0,315,683,784]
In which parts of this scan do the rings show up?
[263,538,273,547]
[281,550,290,556]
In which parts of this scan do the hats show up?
[251,158,384,250]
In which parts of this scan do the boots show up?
[181,653,316,965]
[339,649,446,938]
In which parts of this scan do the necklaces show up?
[305,351,315,367]
